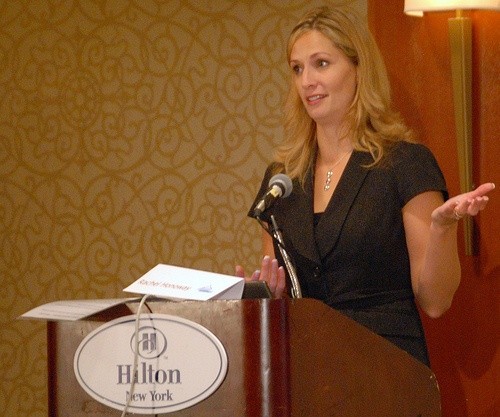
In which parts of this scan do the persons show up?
[226,3,495,373]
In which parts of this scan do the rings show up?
[455,212,464,218]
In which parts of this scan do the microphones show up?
[253,172,293,216]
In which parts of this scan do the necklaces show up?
[322,143,354,192]
